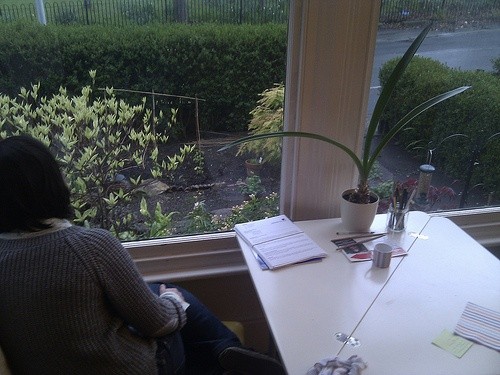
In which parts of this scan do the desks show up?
[235,211,500,375]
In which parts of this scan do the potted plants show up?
[217,21,472,232]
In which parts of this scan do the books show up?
[233,213,327,271]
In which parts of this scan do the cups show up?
[373,243,392,268]
[387,202,409,232]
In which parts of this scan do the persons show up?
[0,135,284,375]
